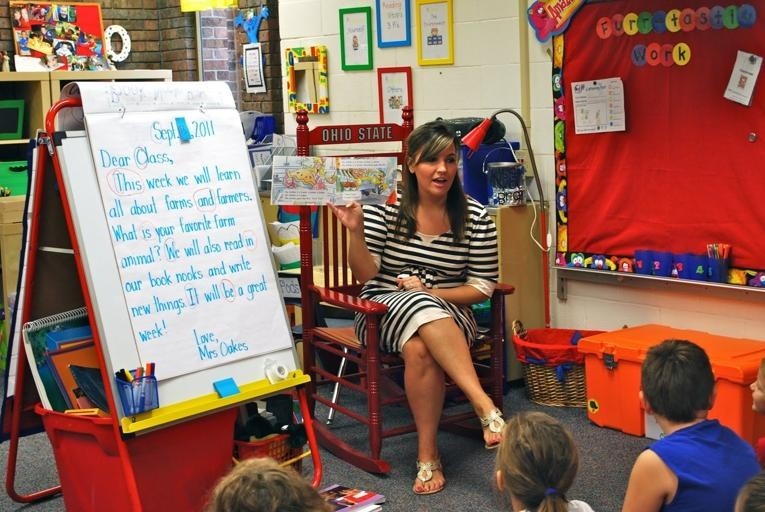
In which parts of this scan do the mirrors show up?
[286,45,328,112]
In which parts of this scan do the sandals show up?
[411,455,449,499]
[477,406,509,450]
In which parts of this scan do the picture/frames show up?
[377,67,414,130]
[415,0,454,65]
[375,0,411,48]
[338,6,373,71]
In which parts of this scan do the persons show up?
[621,340,765,512]
[203,457,336,512]
[749,357,765,468]
[329,121,509,495]
[493,412,595,512]
[733,471,765,512]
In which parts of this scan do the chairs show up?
[295,106,513,476]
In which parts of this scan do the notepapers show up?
[213,377,239,397]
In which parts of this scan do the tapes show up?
[273,364,288,380]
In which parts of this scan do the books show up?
[270,155,398,206]
[316,484,385,512]
[22,306,111,417]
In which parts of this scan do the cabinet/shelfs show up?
[294,62,319,102]
[284,203,543,377]
[0,70,51,347]
[51,70,172,104]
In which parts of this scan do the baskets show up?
[231,422,313,477]
[507,315,632,410]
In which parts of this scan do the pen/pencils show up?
[707,243,731,259]
[114,362,156,416]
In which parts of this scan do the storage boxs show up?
[36,405,234,512]
[645,337,765,466]
[1,161,28,195]
[0,99,25,139]
[580,324,707,436]
